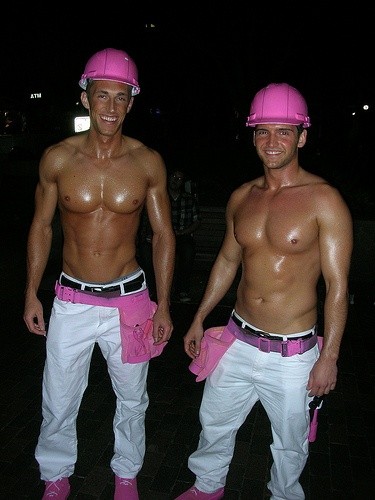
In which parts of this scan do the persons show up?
[176,84,352,500]
[22,47,175,500]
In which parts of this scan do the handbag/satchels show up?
[117,298,167,364]
[188,324,236,382]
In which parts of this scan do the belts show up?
[61,272,144,294]
[230,314,316,341]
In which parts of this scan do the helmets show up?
[78,48,141,95]
[245,82,310,128]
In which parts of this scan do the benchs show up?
[141,204,225,262]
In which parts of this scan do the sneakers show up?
[41,476,71,500]
[174,485,224,500]
[113,474,139,500]
